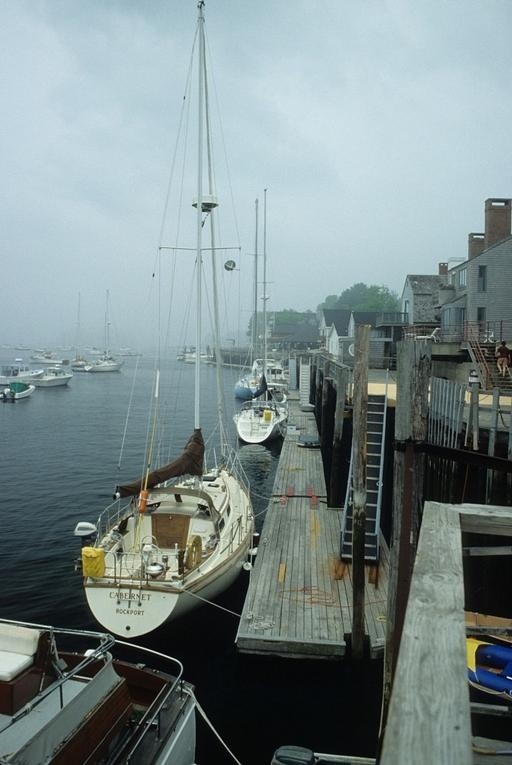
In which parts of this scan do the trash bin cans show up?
[468,369,481,390]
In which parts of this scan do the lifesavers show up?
[184,533,203,571]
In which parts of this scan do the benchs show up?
[0,623,58,716]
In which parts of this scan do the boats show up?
[0,611,197,763]
[175,343,214,364]
[0,343,72,404]
[114,344,144,357]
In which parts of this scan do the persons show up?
[494,340,510,378]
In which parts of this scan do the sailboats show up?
[70,288,124,373]
[233,188,288,445]
[72,2,257,640]
